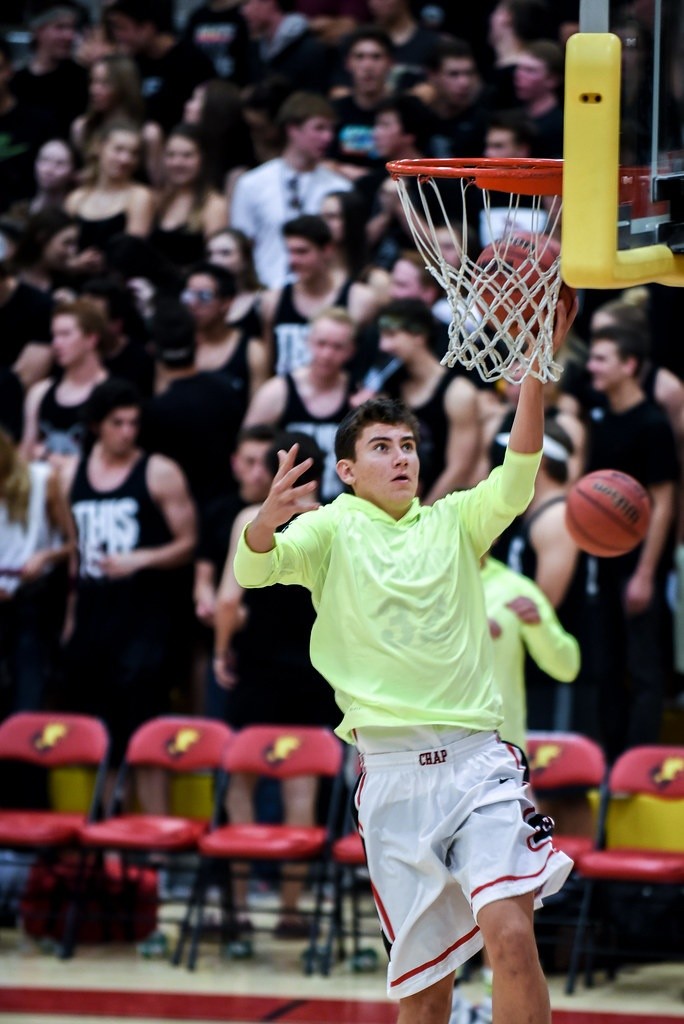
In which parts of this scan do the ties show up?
[288,177,302,215]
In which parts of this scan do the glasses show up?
[182,288,215,304]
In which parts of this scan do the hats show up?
[494,428,569,464]
[87,378,139,424]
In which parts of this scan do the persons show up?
[449,486,578,1024]
[235,296,580,1024]
[1,0,684,974]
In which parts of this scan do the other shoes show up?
[275,919,321,940]
[191,916,256,940]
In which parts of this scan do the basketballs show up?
[565,464,650,558]
[472,235,582,338]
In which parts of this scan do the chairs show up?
[0,709,112,960]
[56,709,237,969]
[523,727,619,987]
[167,727,347,975]
[565,743,684,995]
[317,744,390,974]
